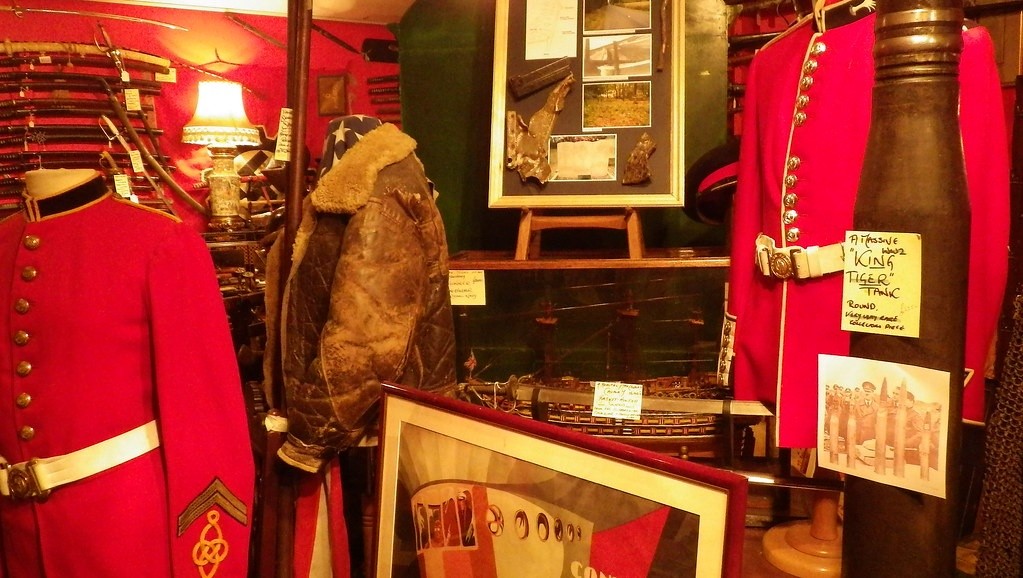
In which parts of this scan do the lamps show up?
[181,79,263,232]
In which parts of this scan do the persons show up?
[824,381,941,471]
[0,170,255,578]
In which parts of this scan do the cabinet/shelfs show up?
[451,249,730,460]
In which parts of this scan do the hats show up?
[862,382,876,391]
[682,142,739,226]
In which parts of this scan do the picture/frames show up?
[368,381,749,578]
[488,0,686,209]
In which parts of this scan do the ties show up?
[866,401,868,405]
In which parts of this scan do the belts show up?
[0,420,160,500]
[753,232,845,279]
